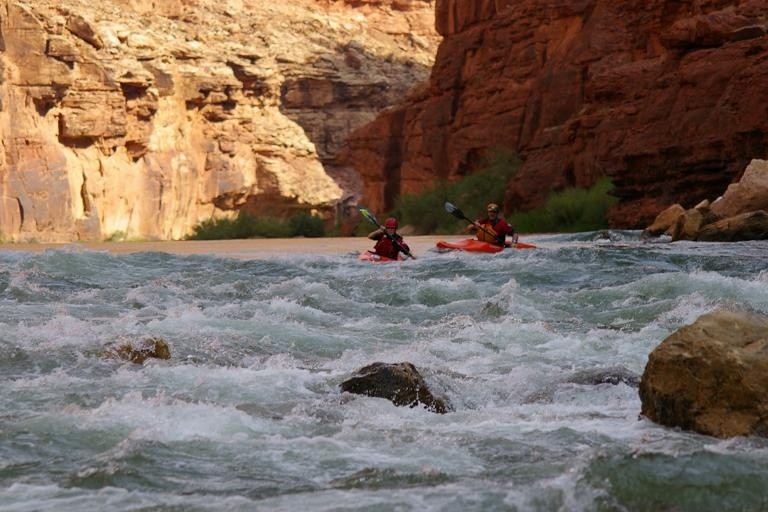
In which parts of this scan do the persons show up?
[467,204,518,247]
[367,218,410,258]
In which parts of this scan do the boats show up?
[436,238,537,253]
[358,248,403,262]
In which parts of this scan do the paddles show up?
[445,202,512,247]
[360,209,416,259]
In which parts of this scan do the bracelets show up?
[512,240,517,243]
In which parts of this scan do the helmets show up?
[384,218,397,229]
[488,204,499,213]
